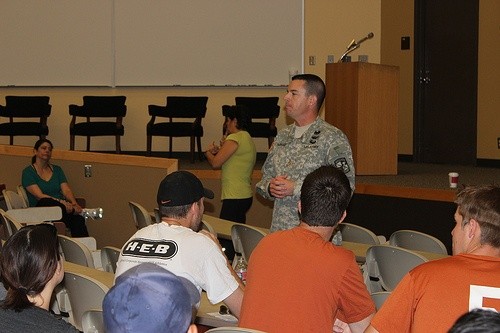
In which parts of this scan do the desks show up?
[149,211,452,262]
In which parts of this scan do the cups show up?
[448,172,459,188]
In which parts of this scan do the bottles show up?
[234,258,248,286]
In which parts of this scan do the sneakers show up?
[83,207,103,221]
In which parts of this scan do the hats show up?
[157,171,214,207]
[102,262,201,333]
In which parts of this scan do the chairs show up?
[0,182,500,332]
[147,96,208,163]
[0,96,52,145]
[69,96,127,154]
[222,97,280,148]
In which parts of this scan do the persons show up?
[0,223,84,333]
[22,138,102,238]
[448,308,500,333]
[239,164,378,333]
[102,262,201,333]
[364,185,500,333]
[255,74,355,246]
[206,105,257,260]
[113,171,246,324]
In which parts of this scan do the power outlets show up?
[358,55,368,63]
[328,56,334,63]
[310,56,315,65]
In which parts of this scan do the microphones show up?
[354,32,374,45]
